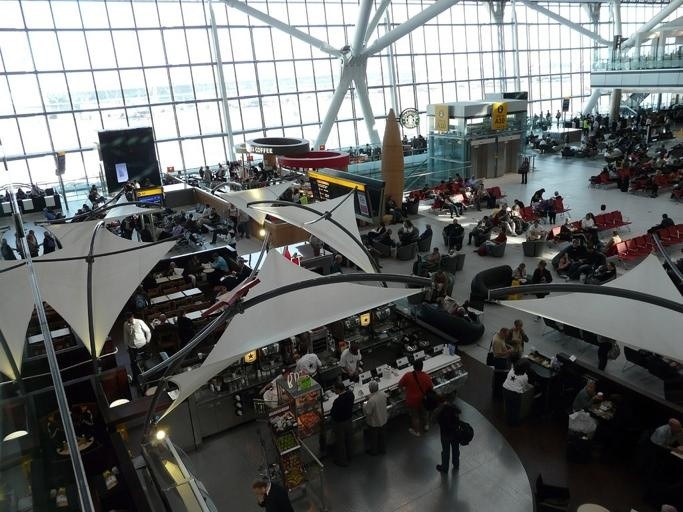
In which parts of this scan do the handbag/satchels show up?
[422,389,438,412]
[487,352,494,366]
[608,347,619,358]
[456,421,474,445]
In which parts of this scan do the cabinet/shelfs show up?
[275,372,323,439]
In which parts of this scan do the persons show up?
[359,172,676,323]
[329,254,343,274]
[489,327,514,402]
[517,101,683,200]
[0,238,16,260]
[249,476,294,511]
[337,341,364,384]
[14,233,25,259]
[325,382,357,468]
[397,357,433,438]
[360,379,390,458]
[16,185,43,212]
[293,343,325,394]
[42,230,55,255]
[427,389,462,473]
[571,376,603,413]
[44,414,61,442]
[75,401,97,434]
[174,157,311,208]
[648,417,683,455]
[344,133,426,160]
[87,184,108,219]
[501,357,534,427]
[506,319,529,369]
[257,366,303,397]
[26,229,39,258]
[41,203,91,224]
[112,201,256,395]
[124,177,156,203]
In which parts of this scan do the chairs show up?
[533,473,570,512]
[141,273,224,330]
[501,381,543,420]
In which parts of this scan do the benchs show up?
[589,144,683,203]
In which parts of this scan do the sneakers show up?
[408,428,420,437]
[424,424,429,430]
[437,465,448,473]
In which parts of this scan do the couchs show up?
[469,262,512,311]
[420,301,484,345]
[403,271,454,305]
